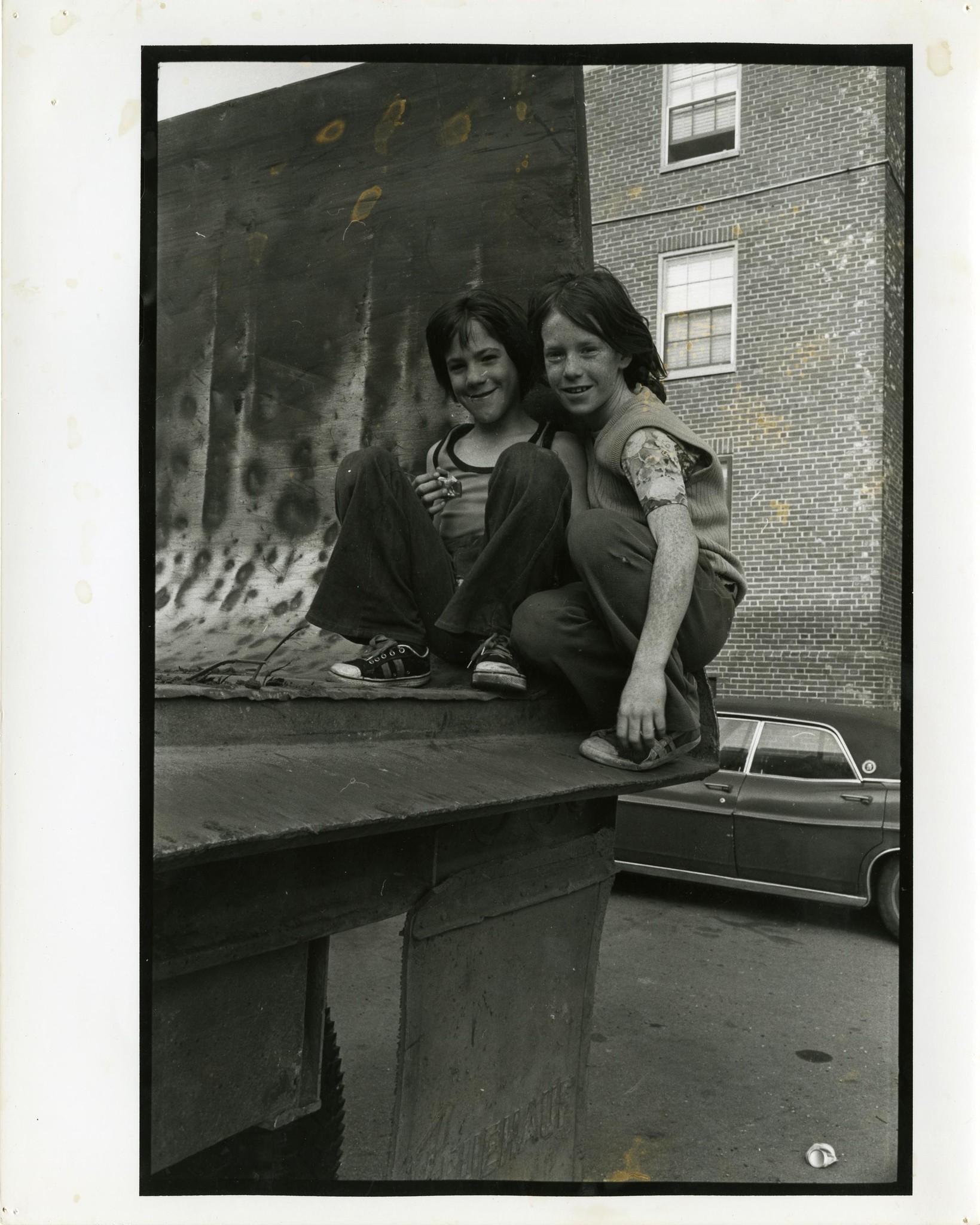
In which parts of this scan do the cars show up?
[613,703,902,935]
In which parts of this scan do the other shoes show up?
[580,718,701,770]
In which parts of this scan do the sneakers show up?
[326,635,432,688]
[467,632,526,692]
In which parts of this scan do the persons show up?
[509,266,747,771]
[305,286,571,692]
